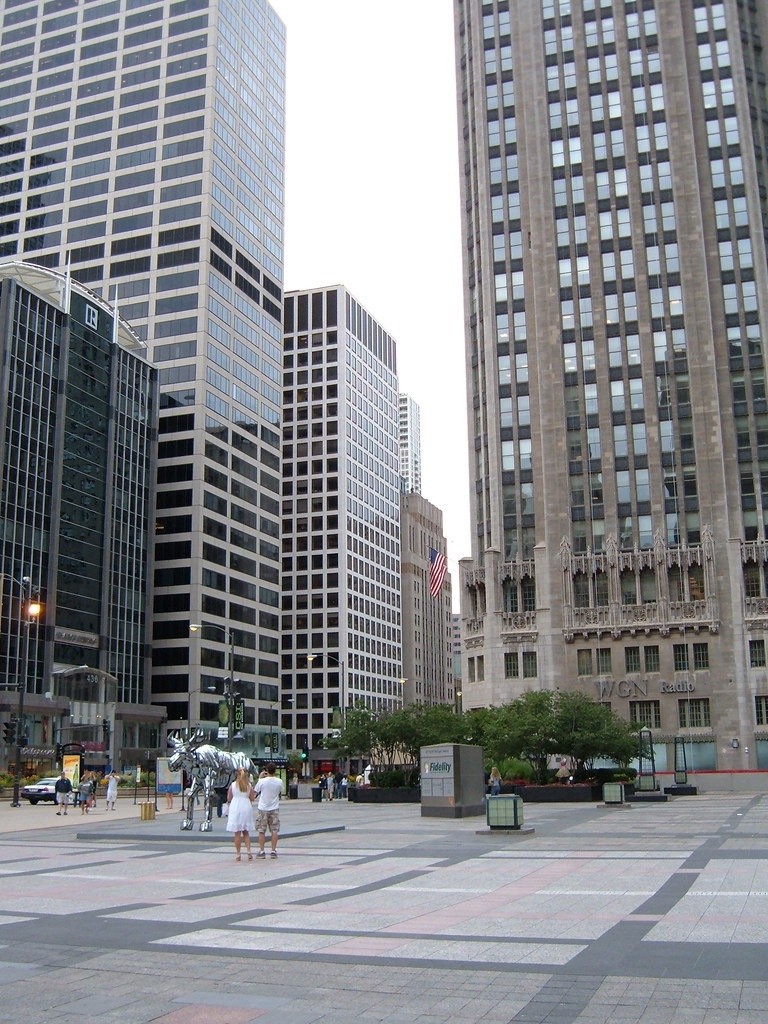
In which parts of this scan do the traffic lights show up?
[2,722,16,744]
[103,718,108,732]
[302,747,308,762]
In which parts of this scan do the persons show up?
[55,772,71,815]
[105,770,120,810]
[319,772,347,800]
[166,793,174,809]
[293,773,298,784]
[356,774,364,786]
[142,780,147,786]
[77,771,102,815]
[489,767,503,796]
[227,768,254,862]
[485,771,490,792]
[254,763,283,858]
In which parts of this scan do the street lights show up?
[306,654,345,774]
[0,573,40,807]
[189,624,238,754]
[269,699,296,759]
[188,685,216,740]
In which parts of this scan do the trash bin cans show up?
[312,788,322,803]
[141,800,155,819]
[289,784,298,799]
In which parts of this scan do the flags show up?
[430,548,447,598]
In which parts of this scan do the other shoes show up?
[249,854,253,860]
[86,809,88,813]
[64,812,68,815]
[236,855,241,862]
[57,812,61,815]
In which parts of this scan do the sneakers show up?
[256,852,266,859]
[271,852,277,858]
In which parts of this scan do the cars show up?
[20,778,59,805]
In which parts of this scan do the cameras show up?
[265,773,267,775]
